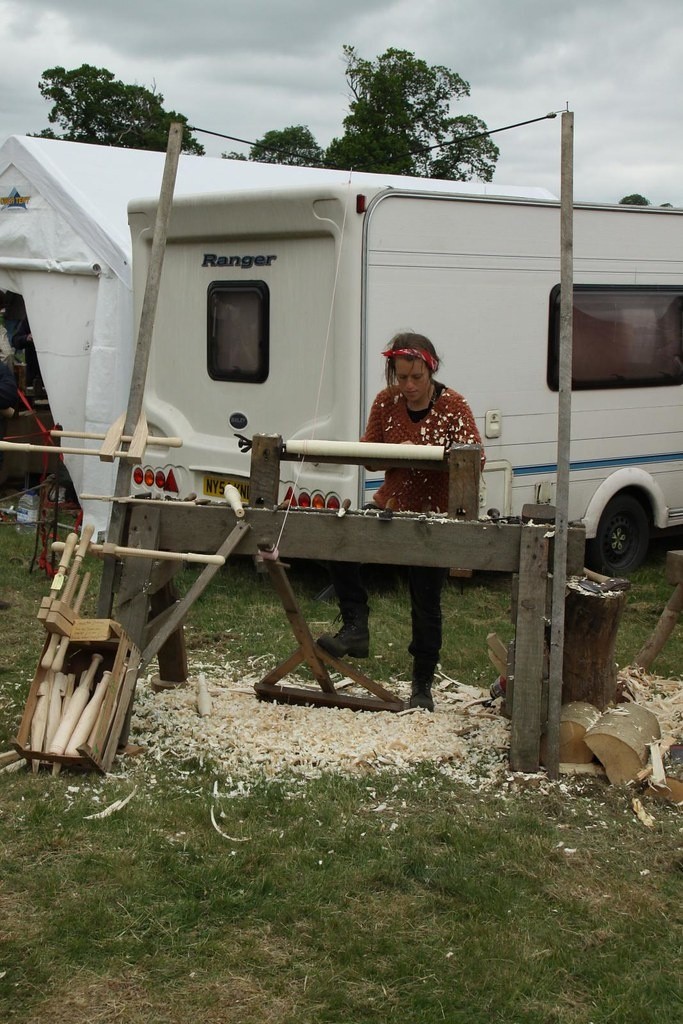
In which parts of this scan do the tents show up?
[0,133,562,542]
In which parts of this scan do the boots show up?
[408,663,436,713]
[317,602,370,658]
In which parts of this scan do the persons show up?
[0,360,19,421]
[11,316,42,390]
[316,334,486,712]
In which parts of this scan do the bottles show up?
[33,376,42,395]
[15,489,40,536]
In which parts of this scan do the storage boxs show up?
[11,619,142,777]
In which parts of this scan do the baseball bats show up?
[44,651,115,756]
[195,673,212,719]
